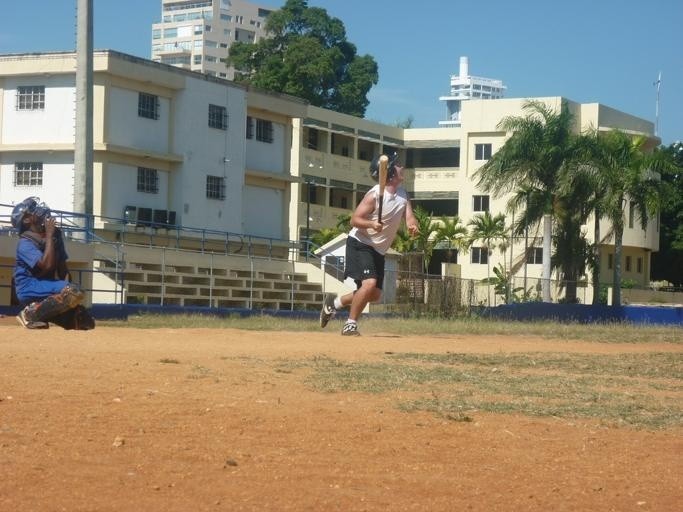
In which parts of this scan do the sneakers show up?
[16,310,36,329]
[320,293,337,328]
[341,323,359,336]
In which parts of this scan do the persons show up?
[320,153,422,337]
[10,197,95,330]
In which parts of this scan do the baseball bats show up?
[378,156,388,222]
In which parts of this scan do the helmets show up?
[11,197,51,233]
[369,154,399,183]
